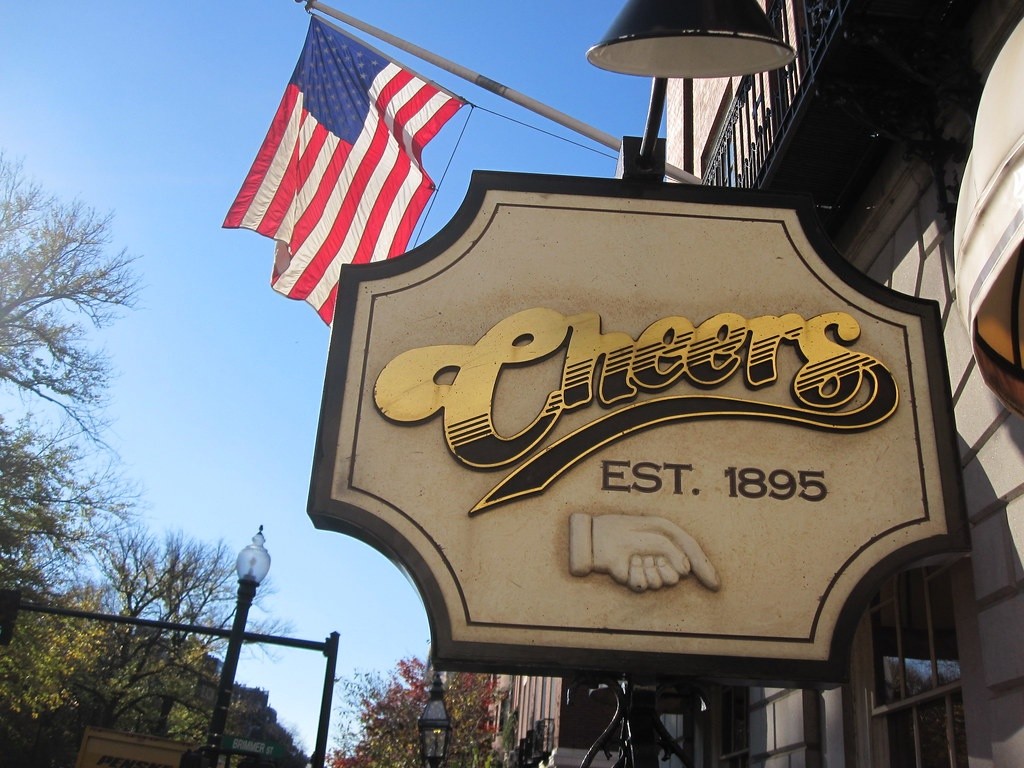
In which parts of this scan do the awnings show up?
[953,15,1024,420]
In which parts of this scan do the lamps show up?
[586,0,796,183]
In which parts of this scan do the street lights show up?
[201,524,271,768]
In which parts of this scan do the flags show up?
[221,15,467,327]
